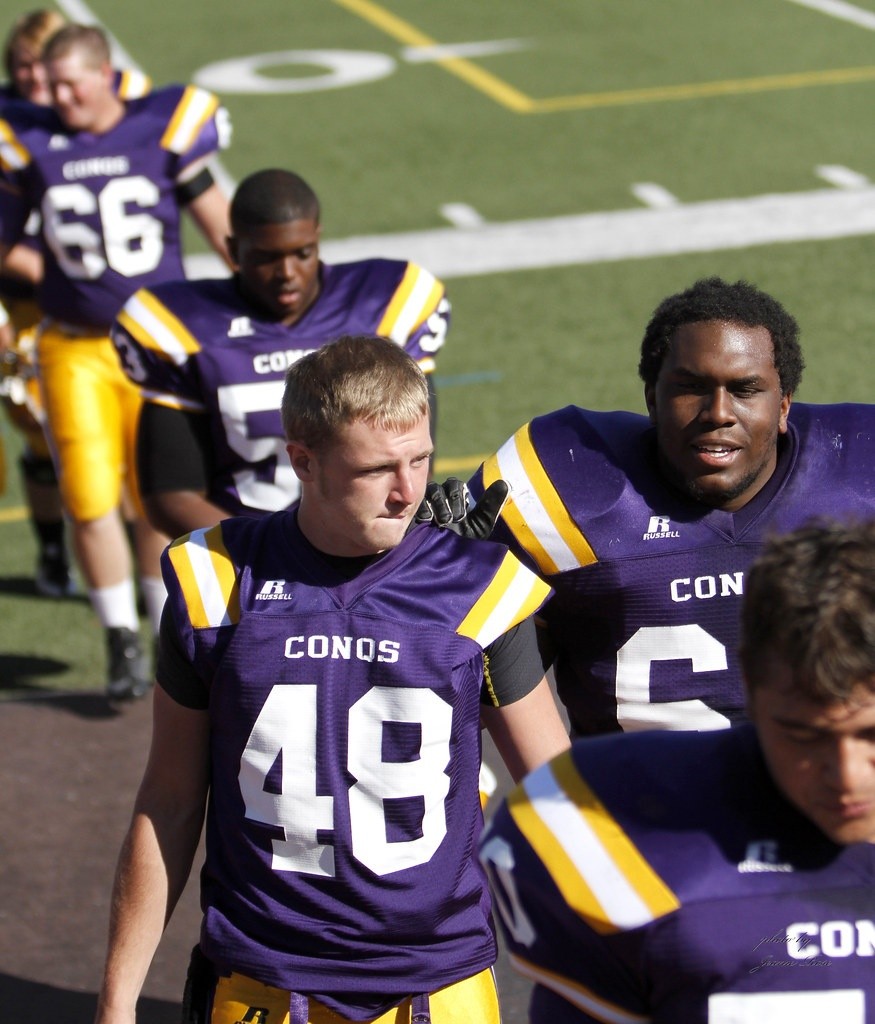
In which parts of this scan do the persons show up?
[94,337,571,1024]
[473,513,874,1024]
[415,276,874,743]
[0,11,239,702]
[111,168,451,543]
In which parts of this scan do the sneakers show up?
[107,627,149,711]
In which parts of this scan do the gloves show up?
[414,477,511,541]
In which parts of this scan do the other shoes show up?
[37,543,71,596]
